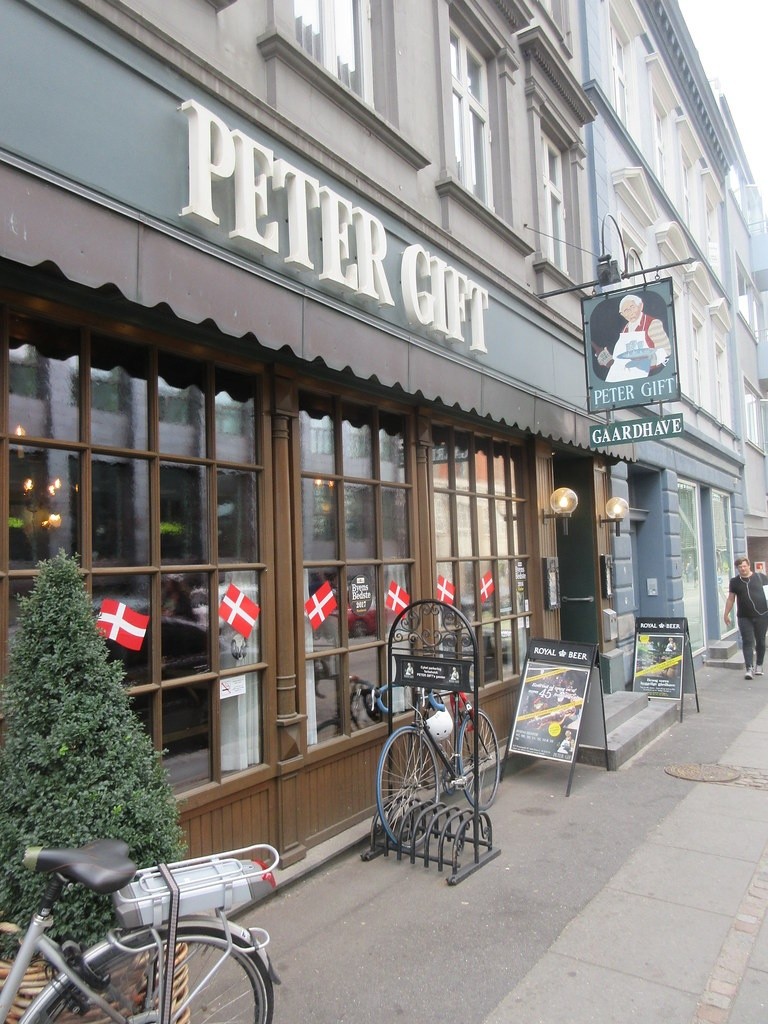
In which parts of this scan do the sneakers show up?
[745,668,754,679]
[755,665,764,675]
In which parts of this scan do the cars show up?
[346,608,396,639]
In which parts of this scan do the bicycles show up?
[369,681,502,852]
[2,835,282,1024]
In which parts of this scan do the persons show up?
[724,557,768,681]
